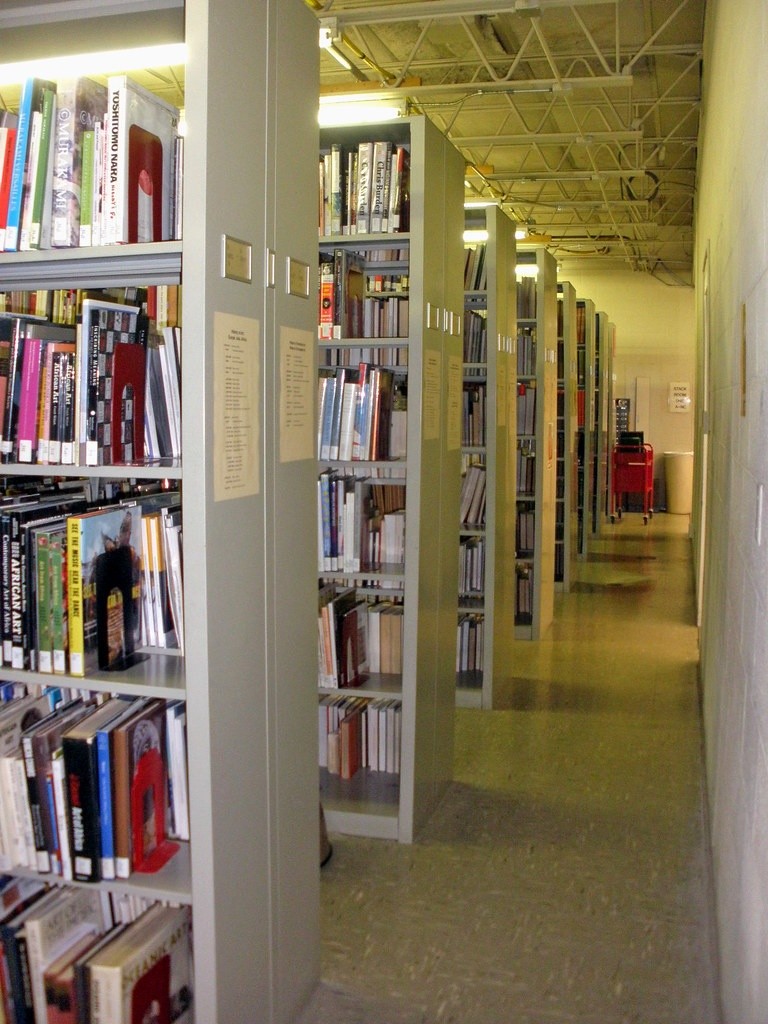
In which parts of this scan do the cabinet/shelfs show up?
[0,0,616,1024]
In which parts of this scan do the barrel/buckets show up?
[663,450,696,515]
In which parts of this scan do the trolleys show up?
[610,443,653,525]
